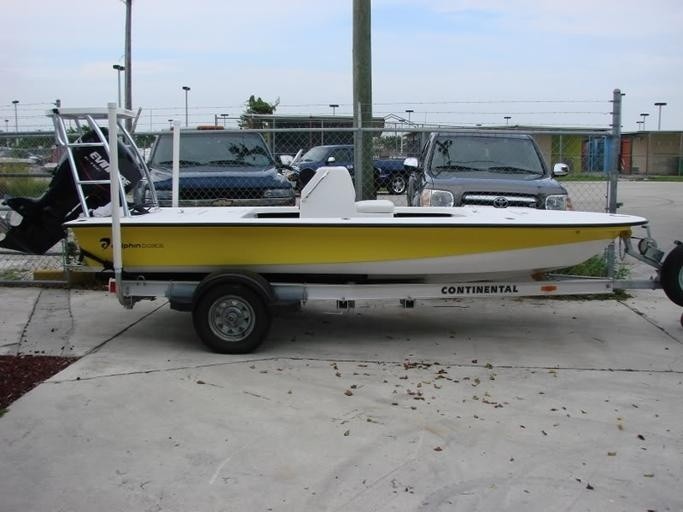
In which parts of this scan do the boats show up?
[0,103,649,273]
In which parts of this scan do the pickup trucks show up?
[283,144,404,194]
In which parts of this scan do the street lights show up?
[504,117,511,124]
[168,119,173,127]
[5,101,20,132]
[183,86,190,127]
[221,113,228,127]
[329,104,338,117]
[406,110,414,128]
[655,102,667,132]
[636,113,649,132]
[113,65,125,108]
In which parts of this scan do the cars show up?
[134,127,295,207]
[404,131,572,211]
